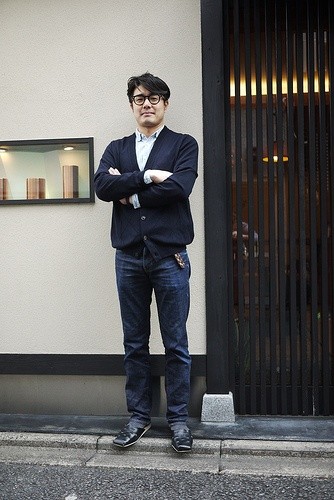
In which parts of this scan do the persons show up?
[93,71,198,452]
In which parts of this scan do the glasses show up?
[132,94,161,106]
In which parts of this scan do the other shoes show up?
[113,420,152,448]
[168,419,193,452]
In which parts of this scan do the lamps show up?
[263,143,288,163]
